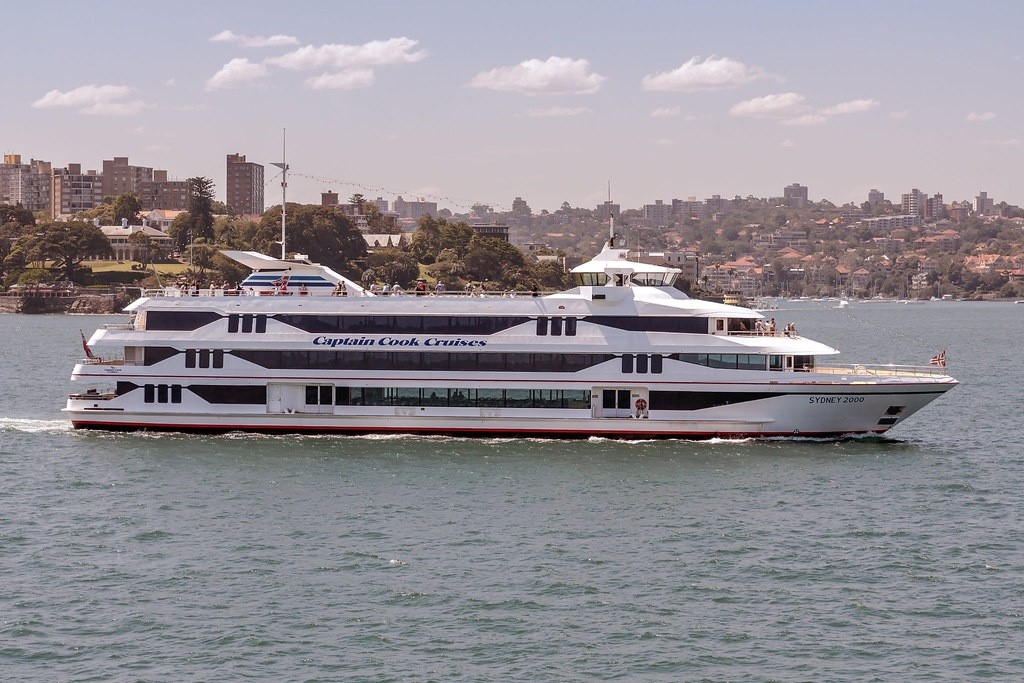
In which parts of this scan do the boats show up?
[61,126,960,443]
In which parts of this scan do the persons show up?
[357,390,559,407]
[465,278,488,298]
[435,280,445,296]
[382,281,391,296]
[784,322,795,337]
[735,321,746,331]
[754,318,776,334]
[175,279,255,297]
[415,281,426,296]
[299,283,308,295]
[531,284,538,297]
[370,279,378,296]
[392,282,404,296]
[336,281,347,296]
[501,288,517,297]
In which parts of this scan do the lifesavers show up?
[635,398,647,410]
